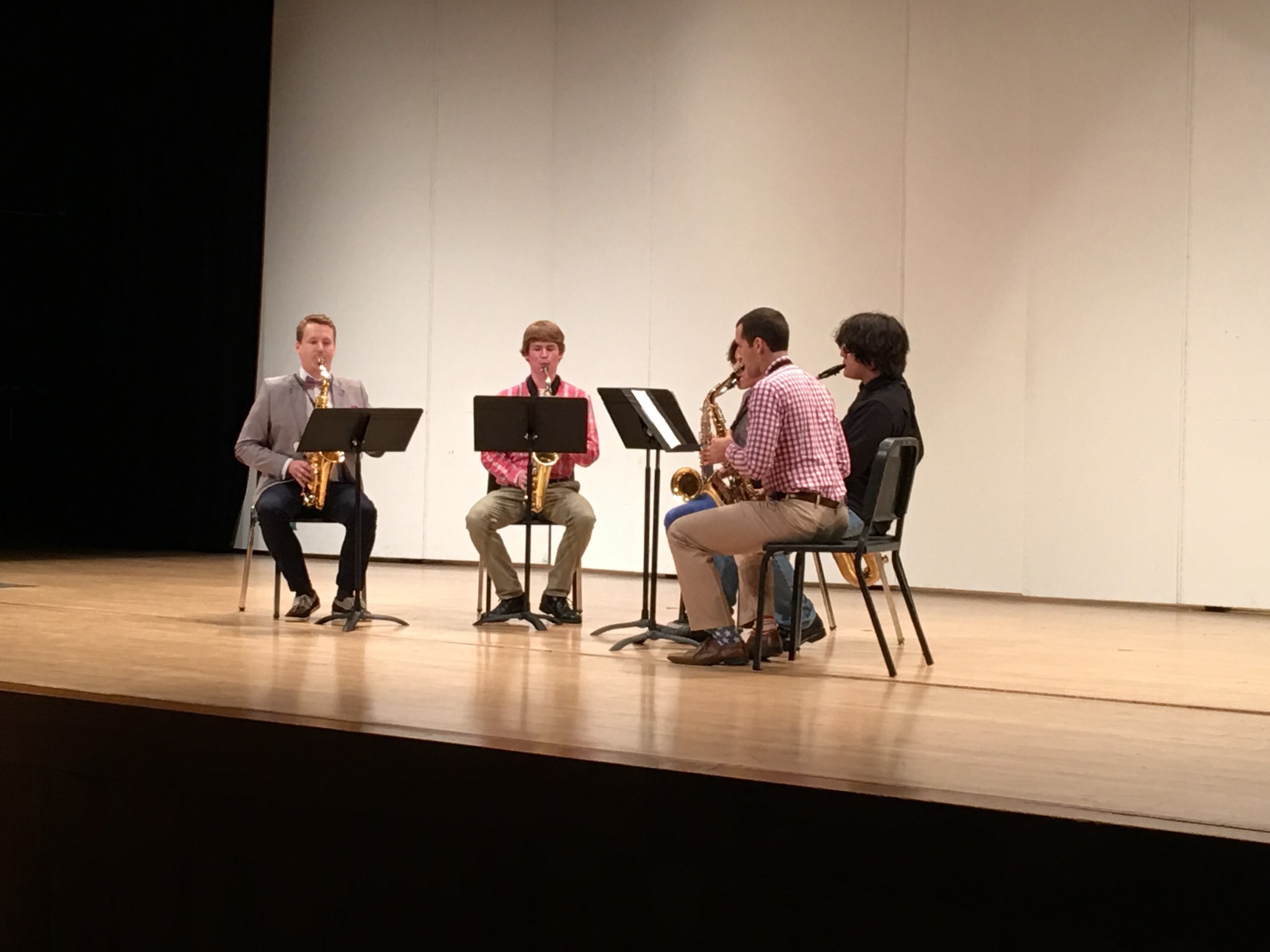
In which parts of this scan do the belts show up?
[549,478,569,484]
[771,490,840,509]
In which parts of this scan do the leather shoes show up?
[539,591,581,623]
[778,612,826,652]
[481,593,524,621]
[746,630,784,657]
[667,637,750,665]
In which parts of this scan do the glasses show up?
[842,347,853,354]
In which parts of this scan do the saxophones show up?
[299,355,346,510]
[670,376,740,502]
[812,362,889,588]
[701,364,764,507]
[522,362,560,513]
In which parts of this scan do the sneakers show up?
[332,595,372,621]
[284,589,321,622]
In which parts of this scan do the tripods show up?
[472,394,588,632]
[591,386,701,652]
[294,408,424,631]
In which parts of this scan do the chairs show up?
[477,468,583,617]
[238,463,367,619]
[676,439,935,677]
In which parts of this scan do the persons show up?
[235,314,378,623]
[465,320,600,625]
[675,311,925,652]
[665,338,765,531]
[663,307,852,666]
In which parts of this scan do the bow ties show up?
[305,376,322,388]
